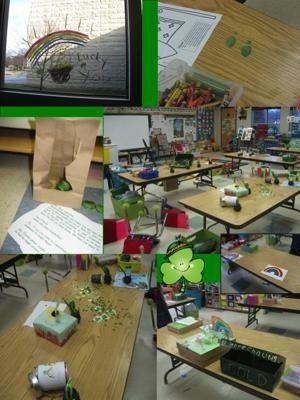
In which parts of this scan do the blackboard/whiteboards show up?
[103,112,152,152]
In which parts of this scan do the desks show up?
[0,146,300,400]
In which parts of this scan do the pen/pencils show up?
[158,79,233,109]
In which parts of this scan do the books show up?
[176,330,236,367]
[168,315,201,334]
